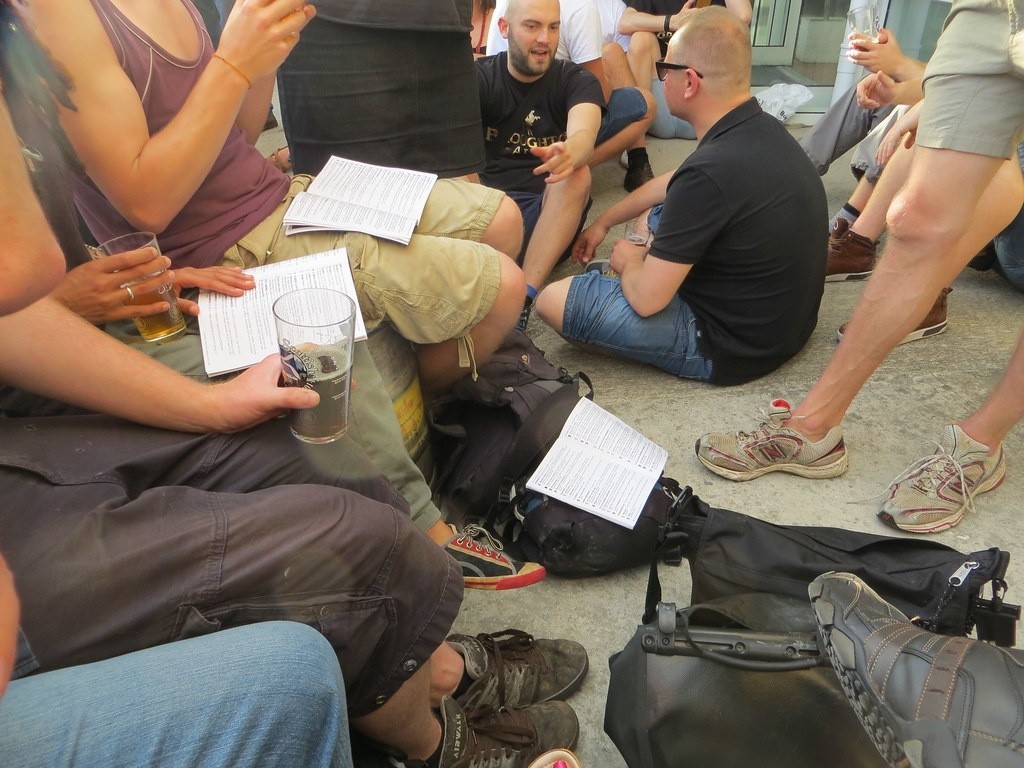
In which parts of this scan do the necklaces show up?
[476,9,487,53]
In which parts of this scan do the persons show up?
[695,0,1024,536]
[0,0,761,768]
[535,6,829,387]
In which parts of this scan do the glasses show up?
[655,57,703,81]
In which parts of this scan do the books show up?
[198,246,368,378]
[527,395,668,530]
[282,155,438,246]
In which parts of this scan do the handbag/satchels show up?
[604,483,1021,768]
[445,330,685,579]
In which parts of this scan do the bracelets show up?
[212,54,253,90]
[663,14,672,32]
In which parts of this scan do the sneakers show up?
[845,425,1005,534]
[400,695,580,768]
[439,522,544,590]
[826,216,880,281]
[808,570,1024,768]
[695,399,849,480]
[836,285,953,348]
[446,627,589,716]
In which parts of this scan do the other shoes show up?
[620,149,628,166]
[623,160,654,192]
[584,259,621,279]
[514,303,531,334]
[527,748,581,768]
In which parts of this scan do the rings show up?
[125,285,134,302]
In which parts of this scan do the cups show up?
[847,4,880,44]
[272,288,356,445]
[624,220,653,248]
[93,232,189,346]
